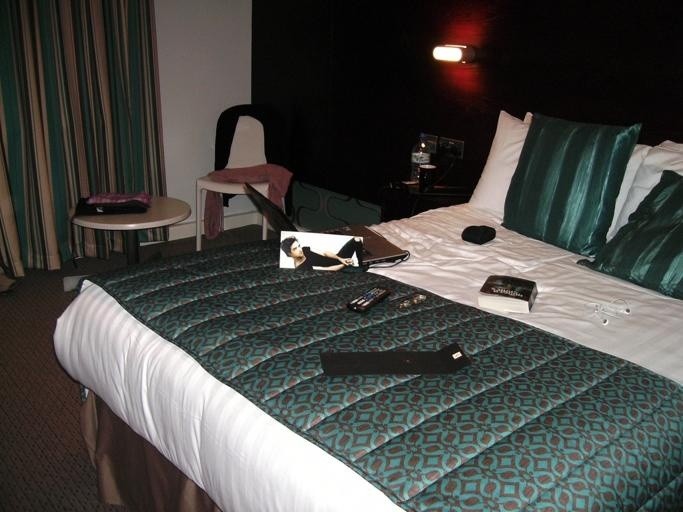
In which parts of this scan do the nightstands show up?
[384,180,468,222]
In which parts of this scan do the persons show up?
[279,237,361,271]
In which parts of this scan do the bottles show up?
[410,132,431,183]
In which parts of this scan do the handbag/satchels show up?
[75,193,148,215]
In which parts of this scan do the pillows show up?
[469,110,683,300]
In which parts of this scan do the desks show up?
[71,196,191,265]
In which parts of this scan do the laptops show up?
[245,182,407,266]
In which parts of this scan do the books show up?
[476,275,537,314]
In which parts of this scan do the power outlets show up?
[419,133,437,154]
[438,137,464,160]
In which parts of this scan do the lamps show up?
[432,44,476,64]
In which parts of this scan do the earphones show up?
[619,309,631,315]
[597,315,608,325]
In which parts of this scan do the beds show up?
[52,203,683,512]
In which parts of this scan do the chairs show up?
[196,104,285,252]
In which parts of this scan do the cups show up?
[418,165,437,193]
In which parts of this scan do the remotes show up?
[348,287,387,313]
